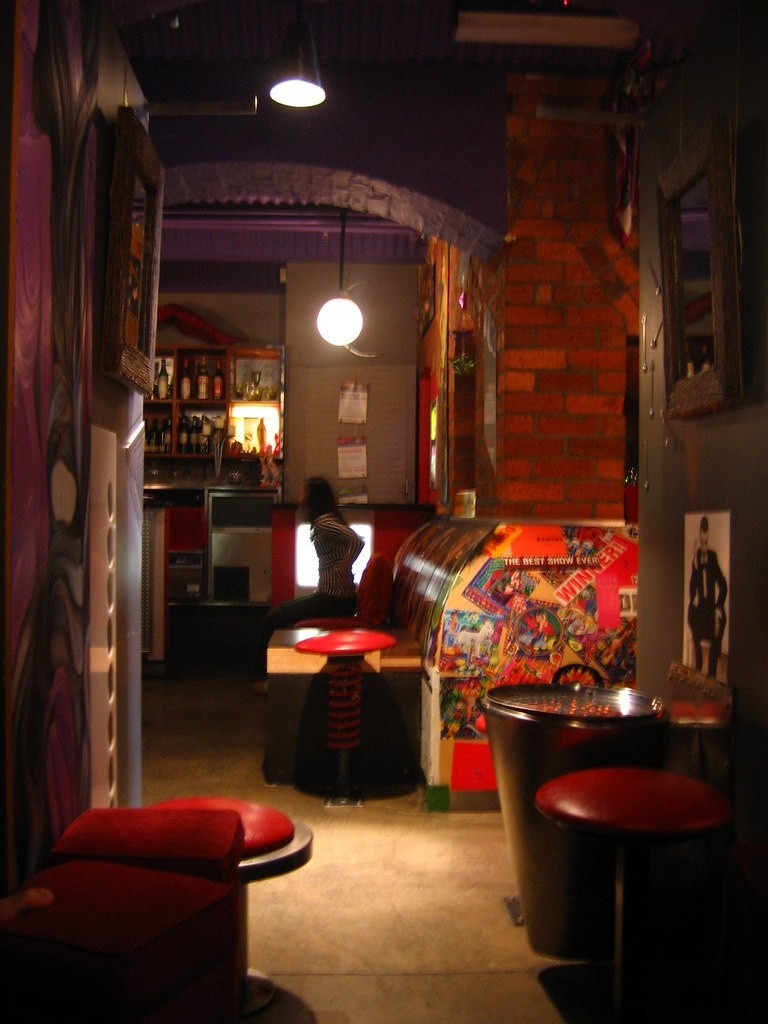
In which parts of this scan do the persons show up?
[244,476,364,696]
[688,518,727,679]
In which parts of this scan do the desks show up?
[267,626,423,674]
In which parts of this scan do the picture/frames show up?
[101,106,165,395]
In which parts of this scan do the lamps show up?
[312,209,366,346]
[269,21,326,108]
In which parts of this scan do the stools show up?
[0,859,235,1024]
[292,628,397,806]
[50,808,245,990]
[534,767,734,1014]
[145,798,314,984]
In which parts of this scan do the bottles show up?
[197,356,209,399]
[145,418,151,446]
[189,416,199,454]
[160,420,167,452]
[178,415,189,454]
[158,360,168,399]
[164,417,171,454]
[211,416,224,454]
[181,358,191,399]
[214,359,224,400]
[199,415,210,454]
[151,419,159,445]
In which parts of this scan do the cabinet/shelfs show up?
[145,304,284,457]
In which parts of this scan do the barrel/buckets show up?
[479,683,661,960]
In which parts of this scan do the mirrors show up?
[657,113,742,417]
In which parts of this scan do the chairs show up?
[292,552,393,626]
